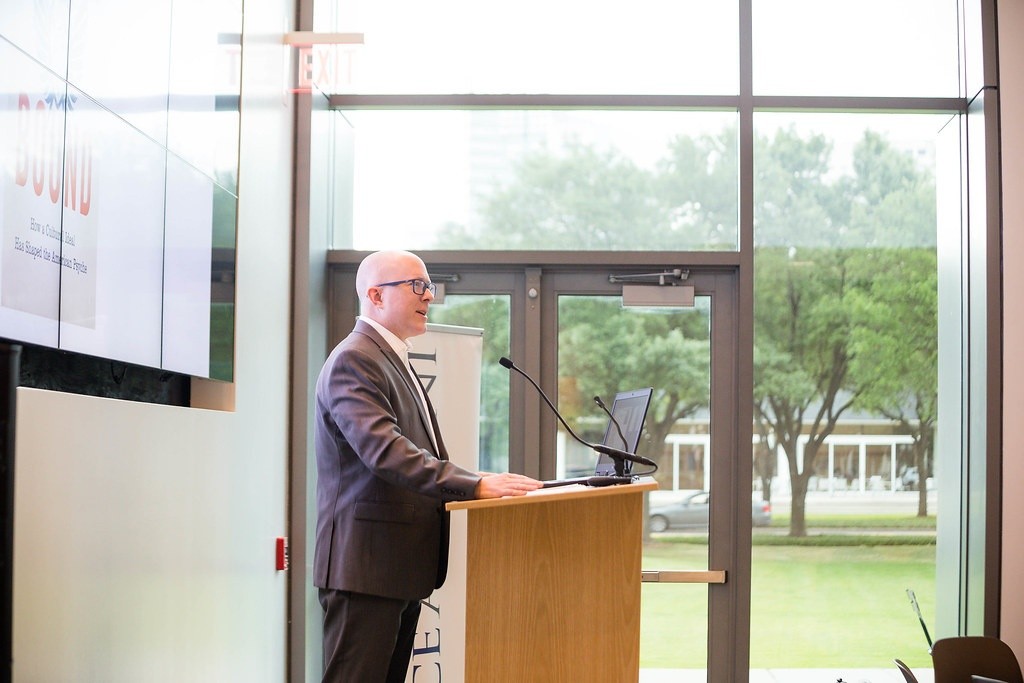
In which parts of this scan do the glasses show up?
[373,278,438,295]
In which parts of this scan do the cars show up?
[648,492,772,535]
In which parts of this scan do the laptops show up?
[538,386,652,489]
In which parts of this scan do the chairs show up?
[892,659,918,683]
[932,636,1024,683]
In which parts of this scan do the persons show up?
[315,249,544,683]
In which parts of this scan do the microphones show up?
[592,392,632,478]
[499,356,656,468]
